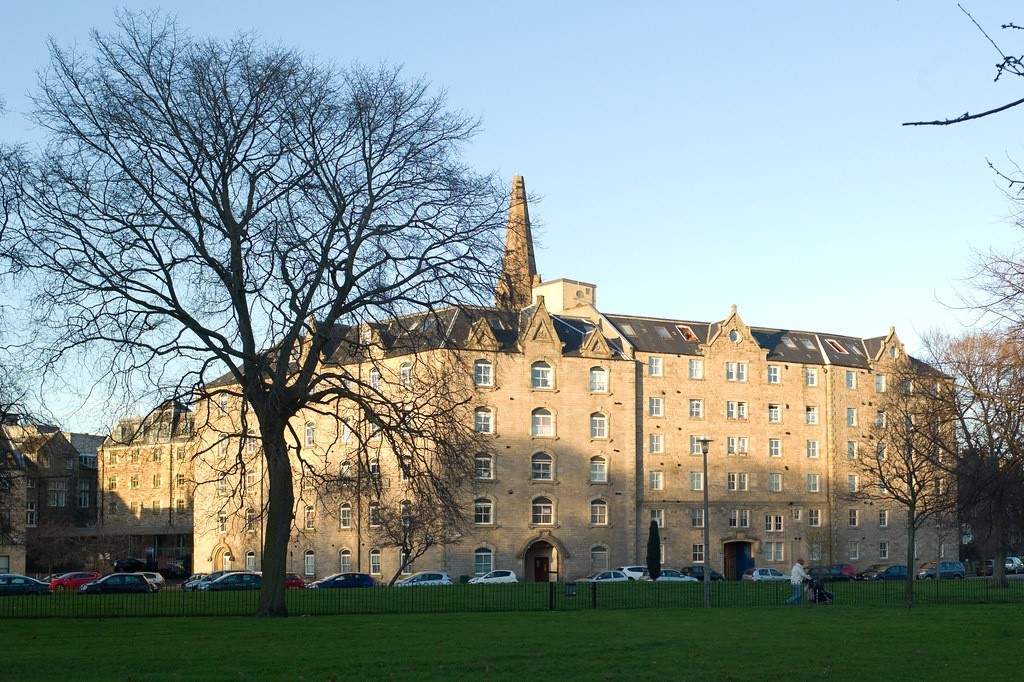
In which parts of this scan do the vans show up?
[916,560,965,580]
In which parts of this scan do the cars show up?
[41,571,102,591]
[77,572,165,593]
[803,562,907,581]
[114,558,145,572]
[467,569,520,584]
[181,568,262,592]
[394,571,454,587]
[741,567,791,584]
[285,571,377,589]
[573,565,724,582]
[0,574,51,595]
[976,556,1024,576]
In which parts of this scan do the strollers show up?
[806,579,832,605]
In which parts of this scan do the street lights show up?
[696,437,714,608]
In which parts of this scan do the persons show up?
[785,558,811,607]
